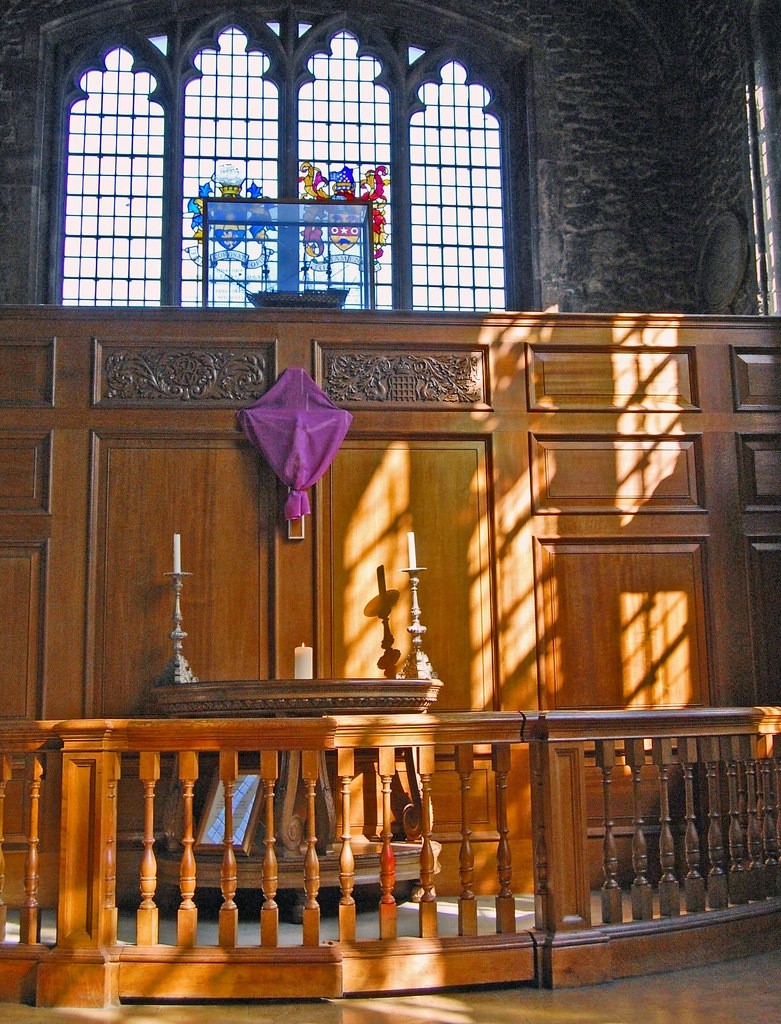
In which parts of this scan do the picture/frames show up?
[191,768,262,856]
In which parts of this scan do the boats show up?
[214,232,351,308]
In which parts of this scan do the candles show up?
[174,534,181,573]
[407,531,417,568]
[294,642,313,679]
